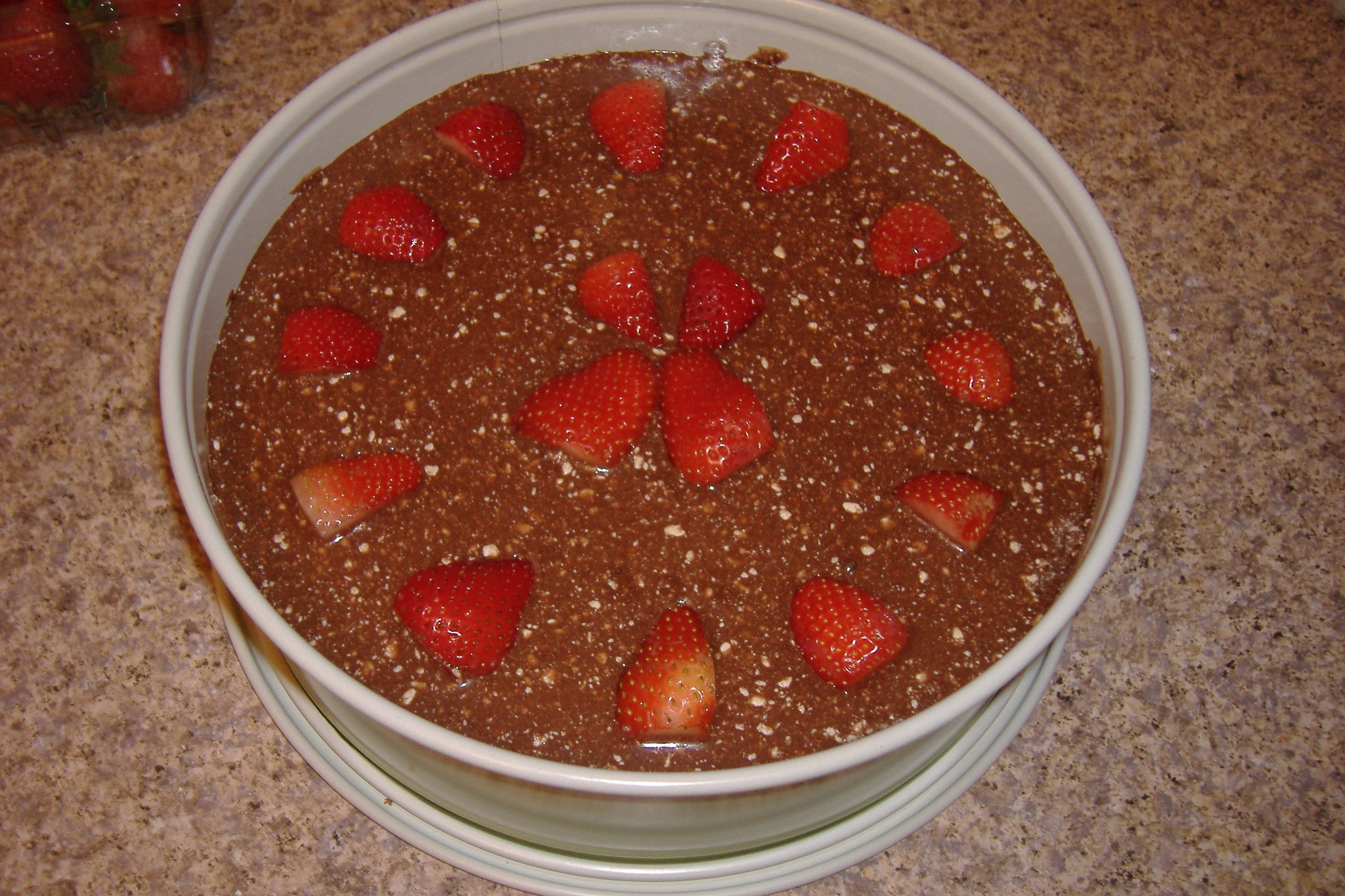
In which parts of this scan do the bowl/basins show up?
[157,0,1154,861]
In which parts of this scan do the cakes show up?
[205,51,1105,777]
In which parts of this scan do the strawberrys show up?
[0,0,207,135]
